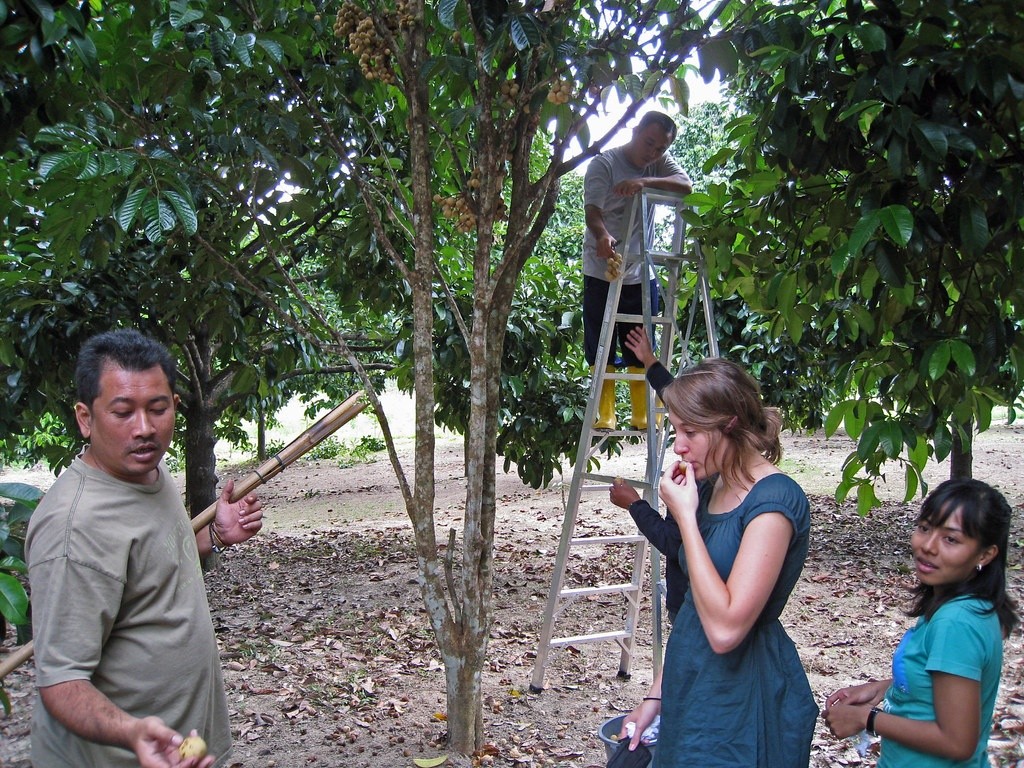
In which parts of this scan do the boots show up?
[590,364,617,430]
[627,366,659,432]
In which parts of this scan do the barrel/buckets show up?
[597,714,661,768]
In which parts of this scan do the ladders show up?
[528,186,721,697]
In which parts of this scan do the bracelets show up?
[208,520,229,555]
[643,697,661,701]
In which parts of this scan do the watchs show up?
[865,707,883,738]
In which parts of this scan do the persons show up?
[581,111,692,432]
[610,325,820,768]
[821,478,1021,768]
[23,327,264,768]
